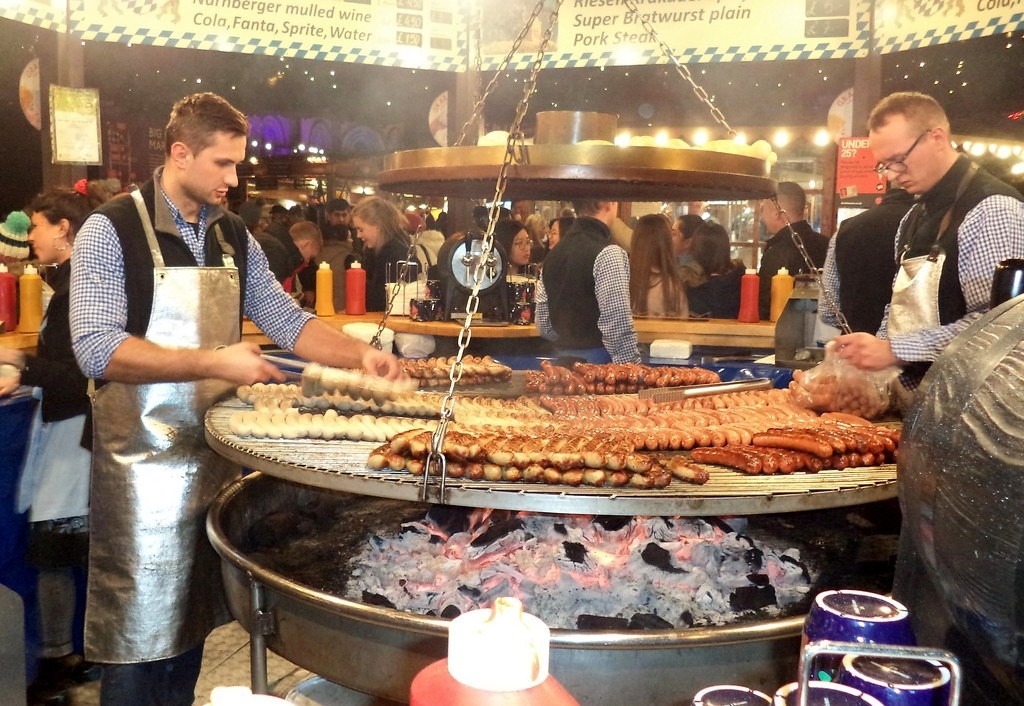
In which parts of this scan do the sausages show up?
[225,354,903,488]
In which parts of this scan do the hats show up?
[0,211,32,264]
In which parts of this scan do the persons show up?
[759,182,831,321]
[0,180,96,706]
[819,180,922,580]
[73,177,538,313]
[404,200,746,320]
[731,199,773,269]
[539,201,642,366]
[831,92,1023,419]
[69,90,408,706]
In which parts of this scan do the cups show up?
[516,303,531,324]
[410,298,443,322]
[689,589,950,706]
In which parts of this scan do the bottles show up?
[796,268,824,288]
[737,265,761,324]
[315,261,336,315]
[410,597,580,706]
[346,260,367,315]
[0,262,43,332]
[770,267,793,323]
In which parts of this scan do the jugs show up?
[426,280,441,299]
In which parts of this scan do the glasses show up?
[514,240,534,249]
[874,129,932,178]
